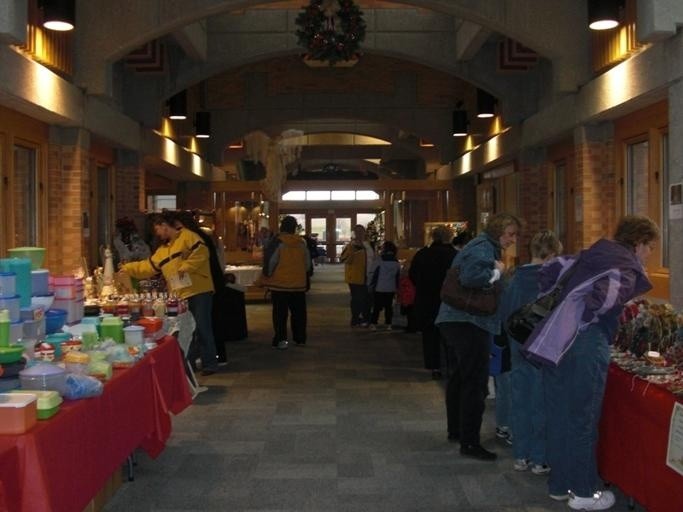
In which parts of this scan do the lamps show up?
[197,111,211,138]
[452,110,467,136]
[42,0,76,32]
[477,91,494,118]
[169,90,188,120]
[587,0,620,30]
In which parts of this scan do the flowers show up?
[292,0,366,69]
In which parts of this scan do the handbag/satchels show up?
[488,335,511,376]
[212,286,248,341]
[506,287,563,344]
[440,264,500,317]
[399,277,417,305]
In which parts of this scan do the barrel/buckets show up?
[0,257,32,308]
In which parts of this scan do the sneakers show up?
[549,493,570,501]
[271,336,288,353]
[382,322,392,331]
[290,335,305,347]
[458,440,497,462]
[195,351,228,366]
[532,461,552,476]
[513,456,534,472]
[432,372,442,380]
[495,424,509,440]
[199,369,215,376]
[367,323,377,332]
[504,432,514,448]
[351,318,369,328]
[567,490,616,511]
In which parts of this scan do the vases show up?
[304,54,359,68]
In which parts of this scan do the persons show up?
[410,229,471,381]
[341,225,374,327]
[264,216,313,351]
[524,214,658,509]
[369,241,401,330]
[119,210,230,376]
[436,214,522,461]
[490,230,563,475]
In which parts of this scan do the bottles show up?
[101,292,188,317]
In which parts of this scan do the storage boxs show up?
[84,465,121,511]
[0,246,163,434]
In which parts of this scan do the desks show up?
[598,353,682,512]
[1,358,153,512]
[225,264,272,302]
[165,312,208,399]
[131,337,192,480]
